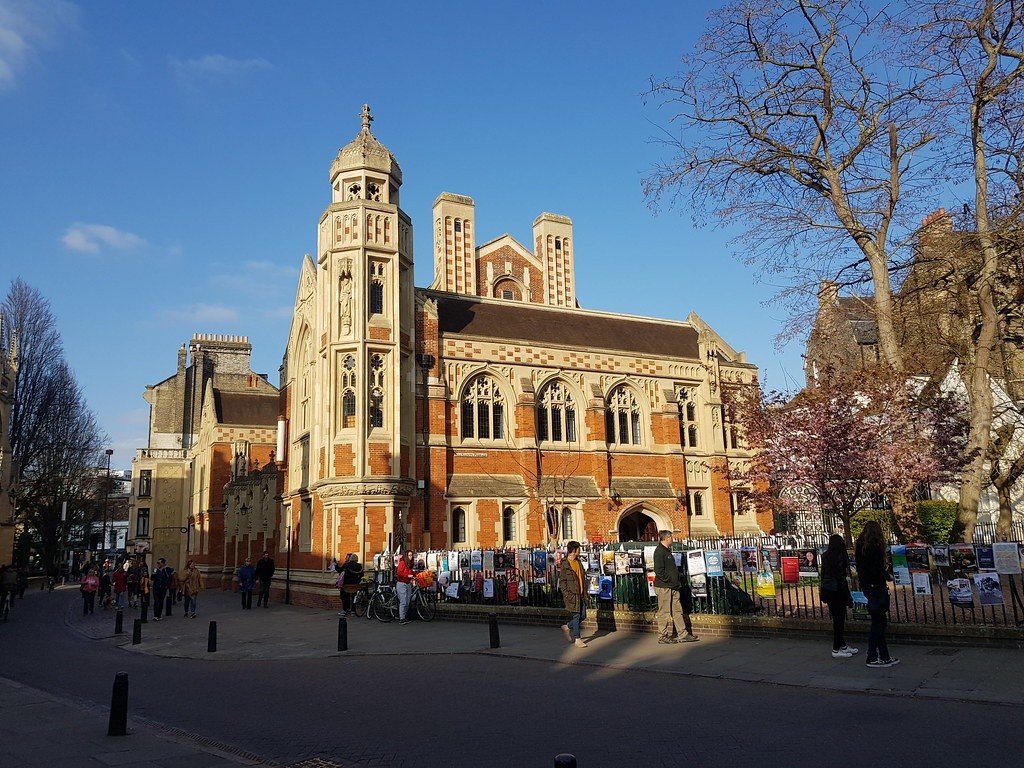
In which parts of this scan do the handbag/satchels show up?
[176,588,184,601]
[416,566,432,587]
[336,571,345,588]
[79,584,85,593]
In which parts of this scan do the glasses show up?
[407,555,413,557]
[157,562,163,564]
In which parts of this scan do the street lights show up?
[99,448,114,598]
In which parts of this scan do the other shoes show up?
[574,638,586,648]
[561,622,573,643]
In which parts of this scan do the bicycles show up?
[353,568,385,617]
[373,575,437,622]
[98,585,114,610]
[2,588,12,621]
[48,574,56,593]
[368,578,412,621]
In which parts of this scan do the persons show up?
[332,554,364,618]
[817,533,858,659]
[805,551,816,568]
[255,551,276,609]
[238,556,256,611]
[396,549,418,627]
[651,530,700,643]
[460,554,468,567]
[336,551,356,616]
[182,560,204,619]
[726,570,760,617]
[496,556,505,568]
[0,558,180,622]
[854,520,900,667]
[558,541,589,648]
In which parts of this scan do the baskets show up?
[417,576,433,588]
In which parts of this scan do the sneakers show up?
[868,658,891,667]
[658,636,676,644]
[84,594,414,625]
[832,650,852,657]
[841,647,860,653]
[879,657,900,665]
[678,634,700,642]
